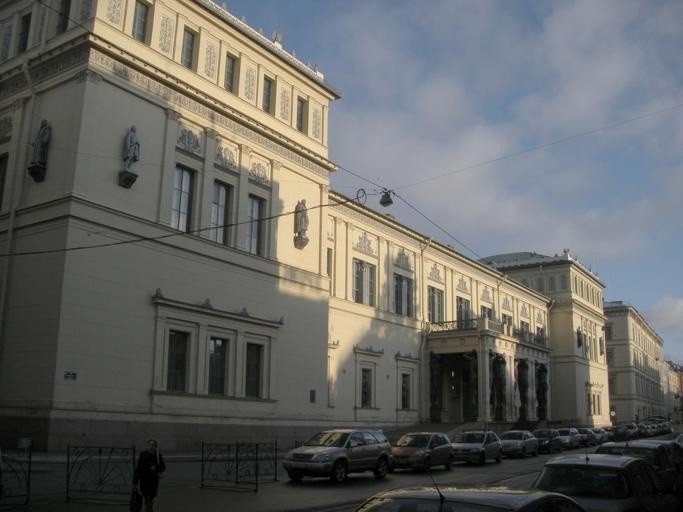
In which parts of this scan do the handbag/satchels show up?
[130,488,143,512]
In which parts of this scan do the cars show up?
[350,470,587,512]
[577,421,671,447]
[530,445,682,511]
[634,441,682,482]
[498,429,539,458]
[392,431,455,474]
[282,428,394,485]
[556,427,580,450]
[450,430,503,466]
[596,440,679,502]
[532,428,562,453]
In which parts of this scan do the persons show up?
[131,437,166,511]
[122,125,141,174]
[293,198,310,239]
[29,116,51,167]
